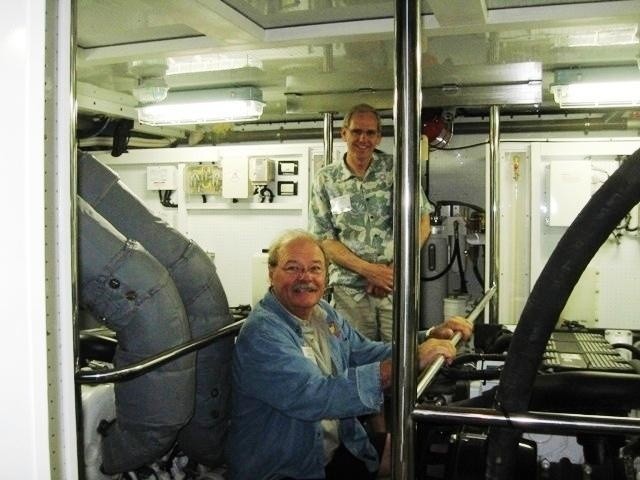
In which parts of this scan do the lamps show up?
[133,85,266,127]
[545,62,639,111]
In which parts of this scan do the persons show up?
[229,228,474,480]
[310,104,435,464]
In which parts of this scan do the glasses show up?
[345,126,380,137]
